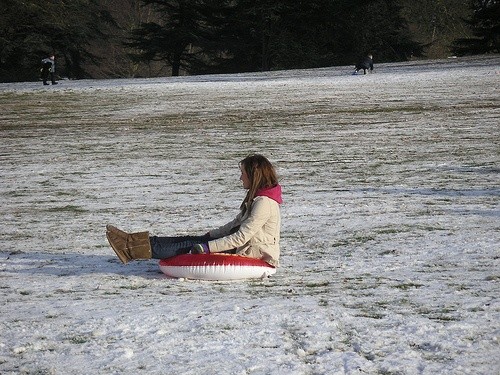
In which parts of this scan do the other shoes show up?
[43,83,49,85]
[52,81,58,84]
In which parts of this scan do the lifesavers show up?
[161,251,277,280]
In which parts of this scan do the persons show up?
[104,154,283,267]
[40,55,58,85]
[354,54,374,74]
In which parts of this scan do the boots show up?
[106,231,151,264]
[106,224,149,240]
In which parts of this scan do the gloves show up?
[190,241,209,254]
